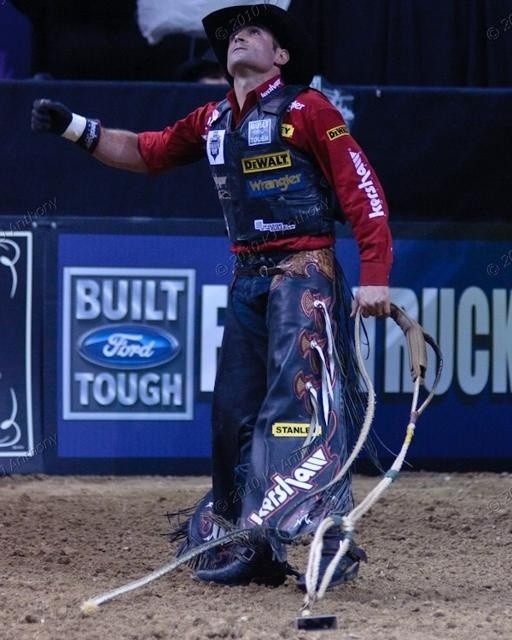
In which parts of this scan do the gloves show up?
[31,97,101,154]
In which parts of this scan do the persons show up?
[32,5,393,592]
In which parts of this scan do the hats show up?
[201,4,306,83]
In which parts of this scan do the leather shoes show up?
[191,546,288,589]
[296,539,361,592]
